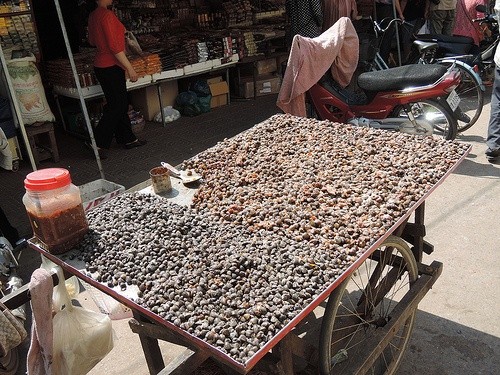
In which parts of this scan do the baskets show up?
[127,104,145,136]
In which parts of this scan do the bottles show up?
[78,73,93,88]
[22,168,89,255]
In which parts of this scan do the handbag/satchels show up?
[51,265,114,375]
[151,77,212,124]
[0,302,27,352]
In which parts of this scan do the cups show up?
[149,167,171,194]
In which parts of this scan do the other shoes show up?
[486,155,500,164]
[84,137,107,160]
[125,138,147,149]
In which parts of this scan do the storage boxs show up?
[254,73,282,97]
[78,179,125,211]
[209,81,229,109]
[130,79,179,122]
[252,58,278,75]
[233,77,255,99]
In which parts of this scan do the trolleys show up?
[0,112,474,375]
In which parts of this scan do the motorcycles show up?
[294,16,472,144]
[404,6,500,87]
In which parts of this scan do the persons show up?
[286,0,358,45]
[397,0,485,72]
[84,0,147,160]
[486,0,500,160]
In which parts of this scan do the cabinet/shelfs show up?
[116,4,211,35]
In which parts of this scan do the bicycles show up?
[348,17,486,133]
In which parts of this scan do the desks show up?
[237,51,289,85]
[53,64,236,139]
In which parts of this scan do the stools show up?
[25,123,59,167]
